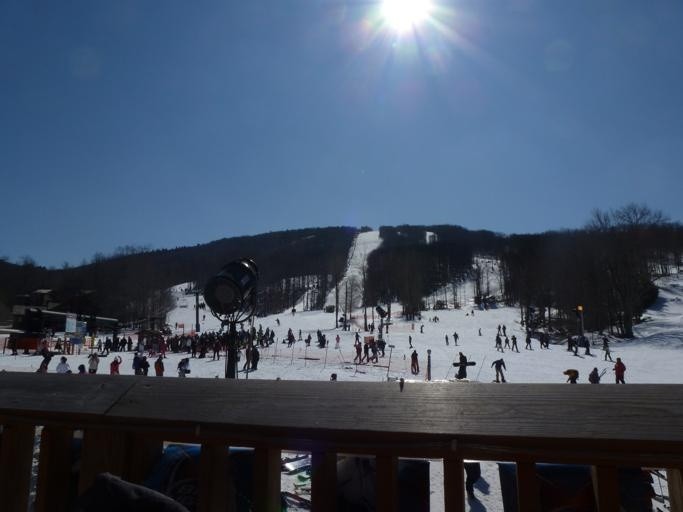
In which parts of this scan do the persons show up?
[495,324,614,362]
[490,359,507,382]
[587,366,602,384]
[335,312,386,364]
[458,352,467,377]
[275,318,280,325]
[453,332,459,346]
[35,324,326,378]
[445,334,450,345]
[612,356,627,385]
[477,328,483,336]
[563,368,579,383]
[175,322,177,328]
[192,302,206,311]
[408,316,439,374]
[202,314,205,321]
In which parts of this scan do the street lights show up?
[373,305,387,347]
[200,256,263,379]
[577,305,585,335]
[385,344,395,376]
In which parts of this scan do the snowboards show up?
[453,362,476,366]
